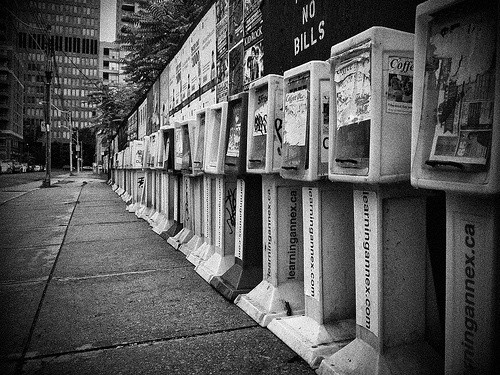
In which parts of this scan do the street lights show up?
[38,100,74,176]
[56,124,80,176]
